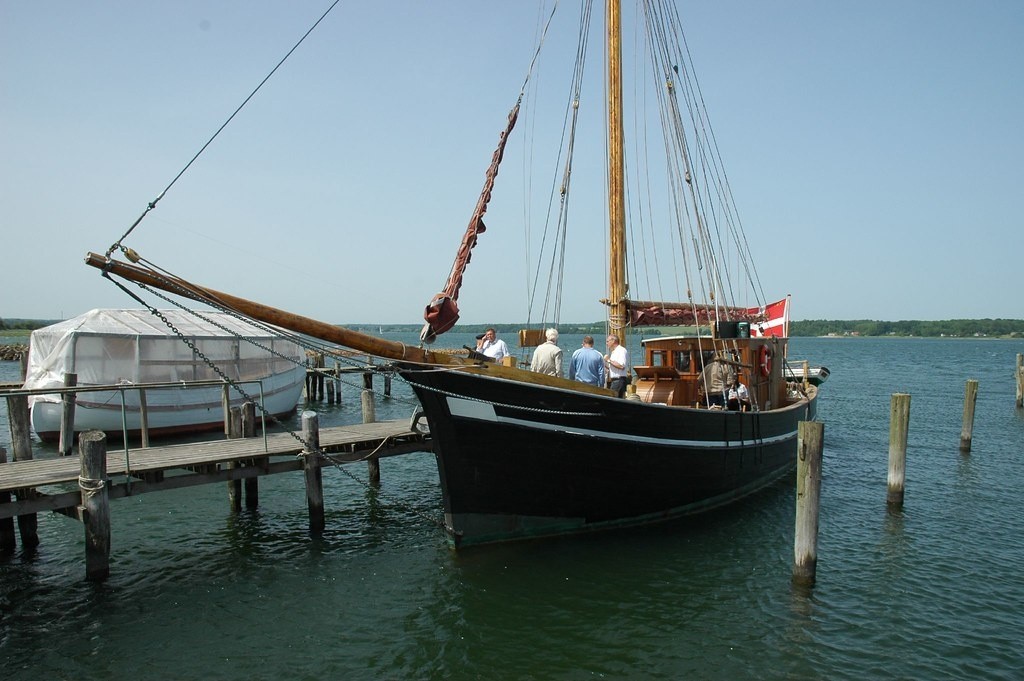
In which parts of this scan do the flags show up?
[747,299,787,337]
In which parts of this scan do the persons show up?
[530,326,566,379]
[697,350,751,412]
[604,334,629,400]
[475,328,509,366]
[567,336,607,387]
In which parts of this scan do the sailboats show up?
[86,3,822,550]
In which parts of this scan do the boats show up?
[23,309,308,443]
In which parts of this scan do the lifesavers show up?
[759,344,772,376]
